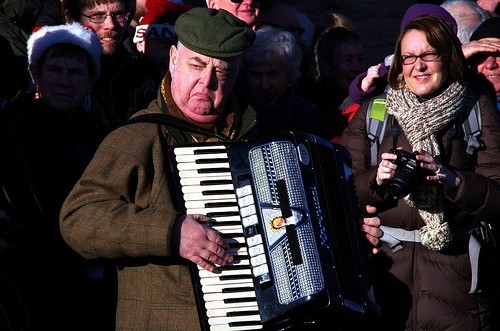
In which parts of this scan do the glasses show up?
[231,0,244,3]
[400,51,441,64]
[79,11,130,23]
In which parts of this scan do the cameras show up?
[387,149,438,200]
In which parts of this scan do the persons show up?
[0,0,500,141]
[59,7,384,331]
[339,14,500,331]
[0,21,117,331]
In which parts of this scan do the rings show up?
[435,168,440,173]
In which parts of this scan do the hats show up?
[400,4,457,34]
[174,7,256,57]
[133,0,193,43]
[27,23,103,86]
